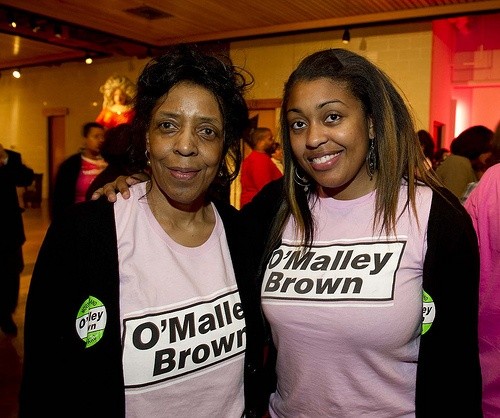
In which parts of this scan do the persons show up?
[463,162,500,418]
[48,71,500,233]
[90,48,484,418]
[0,144,36,336]
[18,45,271,418]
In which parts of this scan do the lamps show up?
[342,29,350,44]
